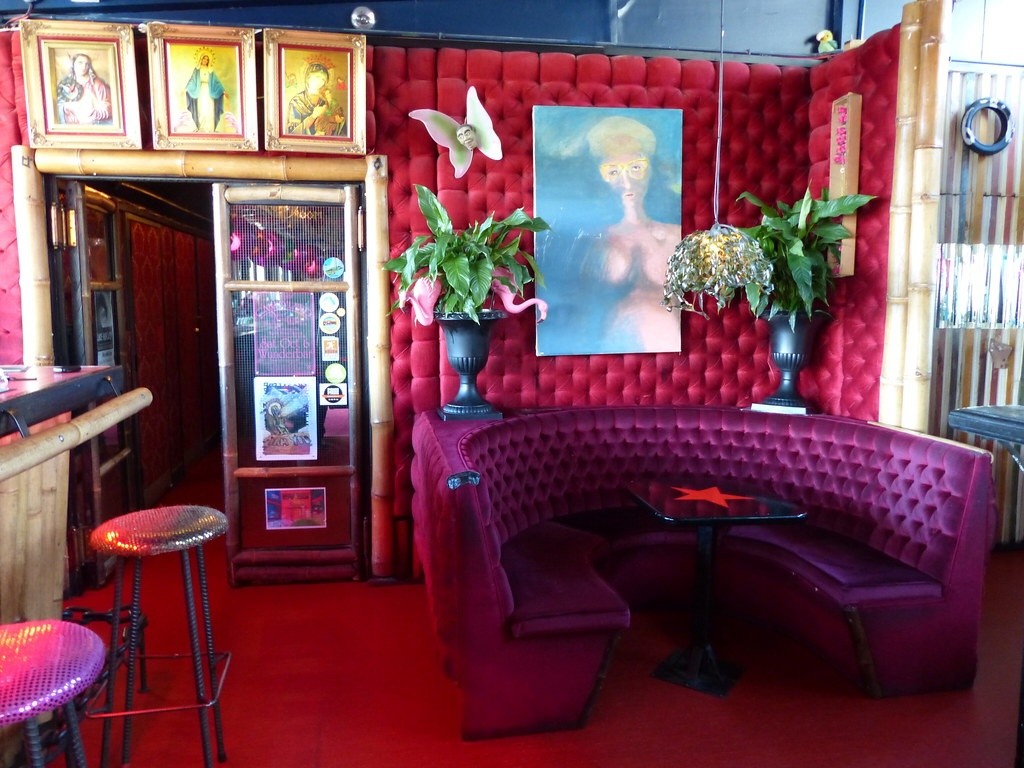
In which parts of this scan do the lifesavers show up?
[960,96,1016,155]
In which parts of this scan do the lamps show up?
[661,0,773,321]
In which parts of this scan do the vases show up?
[432,309,504,421]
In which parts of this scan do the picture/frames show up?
[19,19,142,150]
[253,376,318,460]
[263,28,367,156]
[146,22,259,152]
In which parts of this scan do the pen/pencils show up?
[7,378,37,381]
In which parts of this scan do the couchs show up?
[414,404,995,742]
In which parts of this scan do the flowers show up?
[374,183,548,325]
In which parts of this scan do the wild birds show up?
[398,260,548,326]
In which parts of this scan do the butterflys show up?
[408,85,503,179]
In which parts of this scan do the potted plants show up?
[729,178,879,415]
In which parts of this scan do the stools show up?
[89,505,232,768]
[0,619,106,768]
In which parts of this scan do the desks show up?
[621,476,808,696]
[948,404,1024,474]
